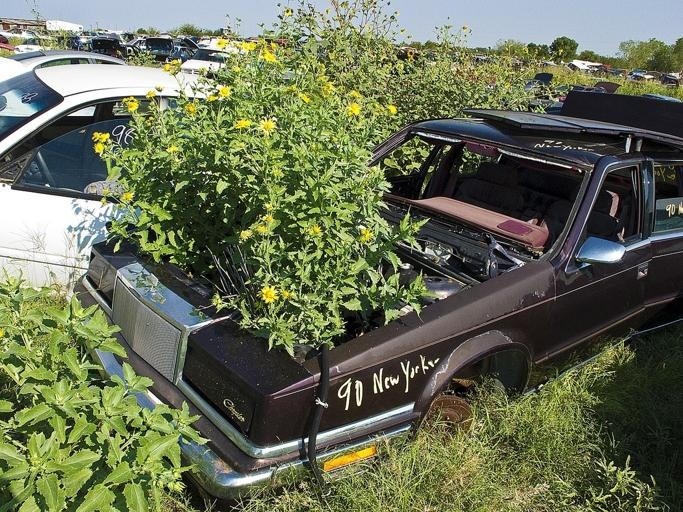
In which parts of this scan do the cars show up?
[56,82,681,506]
[383,46,683,120]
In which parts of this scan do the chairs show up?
[455,162,636,247]
